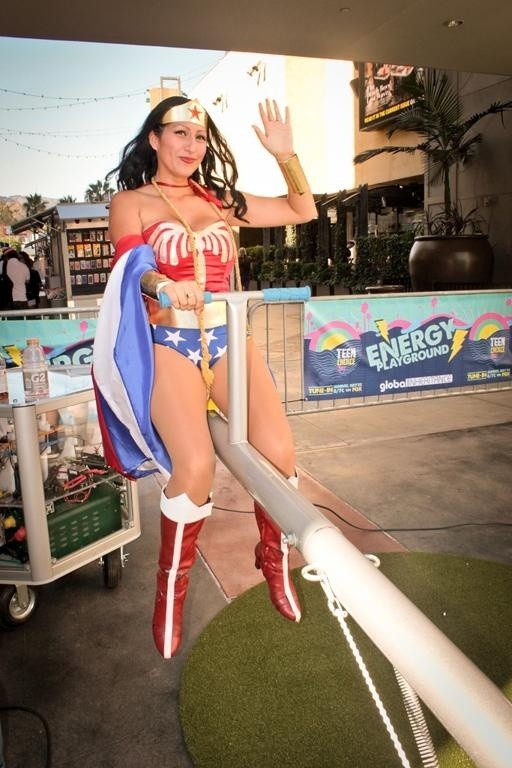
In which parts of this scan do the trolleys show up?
[1,363,143,620]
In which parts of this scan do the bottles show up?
[0,353,10,404]
[22,339,50,403]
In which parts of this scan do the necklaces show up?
[157,179,193,189]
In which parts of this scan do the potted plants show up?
[353,65,512,290]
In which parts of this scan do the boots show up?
[255,466,302,622]
[152,485,214,659]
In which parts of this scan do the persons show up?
[0,246,44,320]
[88,98,317,658]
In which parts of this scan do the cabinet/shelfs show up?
[0,384,145,585]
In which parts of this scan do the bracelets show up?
[280,154,309,198]
[140,268,173,301]
